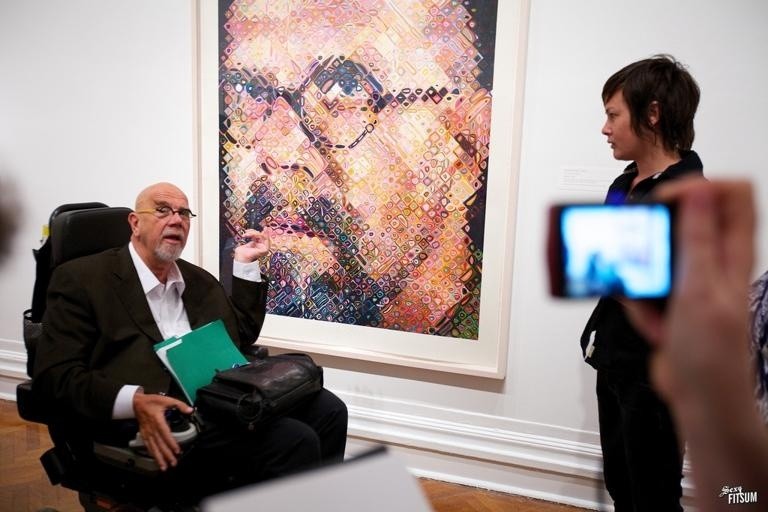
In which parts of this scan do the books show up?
[149,318,251,407]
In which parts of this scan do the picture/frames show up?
[194,0,538,378]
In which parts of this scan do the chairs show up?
[15,203,276,511]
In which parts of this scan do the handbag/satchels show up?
[193,350,323,435]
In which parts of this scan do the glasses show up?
[133,206,197,221]
[220,56,460,150]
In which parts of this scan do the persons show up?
[30,180,351,488]
[613,177,765,510]
[220,1,494,342]
[574,53,717,510]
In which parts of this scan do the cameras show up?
[546,203,676,302]
